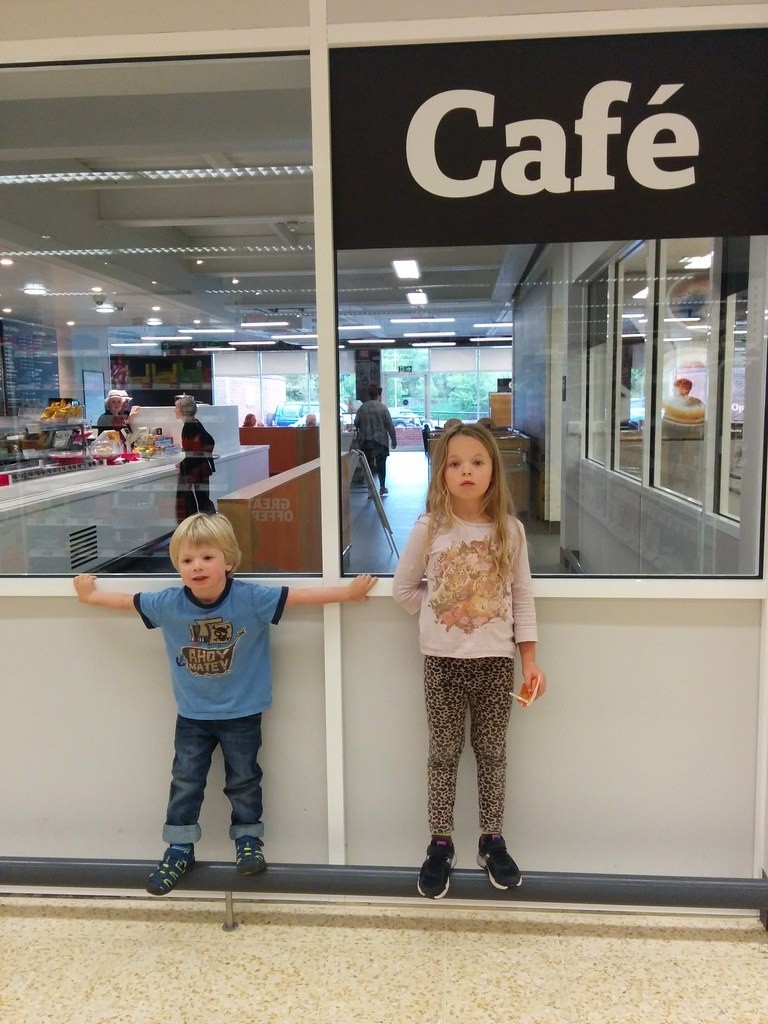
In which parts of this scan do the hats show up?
[105,390,123,403]
[118,390,133,402]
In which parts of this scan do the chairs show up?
[422,425,430,454]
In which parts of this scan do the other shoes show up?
[368,494,372,499]
[380,488,388,495]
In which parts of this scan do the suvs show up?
[266,401,356,426]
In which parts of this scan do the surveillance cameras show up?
[113,302,127,311]
[91,295,106,305]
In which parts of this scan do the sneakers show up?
[235,836,266,876]
[146,843,194,896]
[475,835,522,889]
[417,841,457,899]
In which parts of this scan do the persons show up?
[354,387,397,497]
[74,513,379,896]
[306,414,316,426]
[96,390,140,436]
[174,398,216,516]
[242,414,257,427]
[669,379,705,408]
[392,425,546,900]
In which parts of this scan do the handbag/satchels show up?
[350,427,363,455]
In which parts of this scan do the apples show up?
[134,447,156,453]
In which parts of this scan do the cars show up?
[388,407,433,428]
